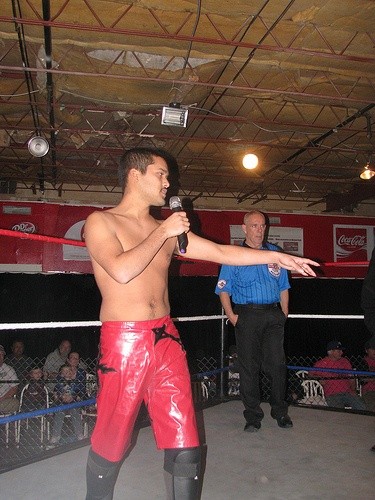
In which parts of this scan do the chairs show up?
[192,382,209,402]
[0,383,98,448]
[296,370,362,408]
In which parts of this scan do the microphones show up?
[170,196,189,254]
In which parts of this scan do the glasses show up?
[246,224,266,231]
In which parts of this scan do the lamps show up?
[27,135,49,158]
[360,165,375,180]
[161,102,189,129]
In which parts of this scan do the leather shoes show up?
[244,410,265,433]
[270,408,293,429]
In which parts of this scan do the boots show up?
[86,447,124,500]
[163,444,207,500]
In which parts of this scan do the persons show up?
[214,211,295,431]
[85,149,321,500]
[0,249,375,451]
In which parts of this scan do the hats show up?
[0,345,7,355]
[229,344,236,354]
[364,336,375,349]
[327,340,347,351]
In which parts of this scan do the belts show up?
[234,303,278,310]
